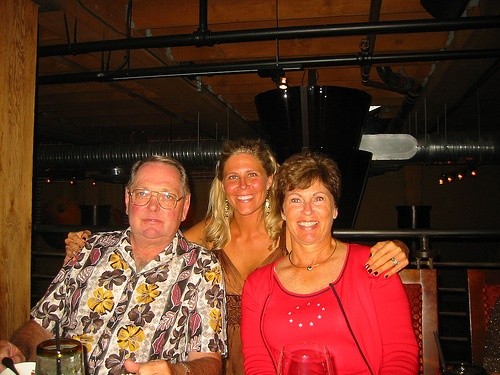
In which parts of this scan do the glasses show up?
[129,189,185,210]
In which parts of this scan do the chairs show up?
[399,269,441,375]
[468,269,500,375]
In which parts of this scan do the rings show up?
[392,258,397,265]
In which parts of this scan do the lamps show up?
[258,65,288,90]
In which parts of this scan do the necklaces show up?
[289,238,337,271]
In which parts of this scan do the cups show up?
[440,361,487,375]
[277,341,333,375]
[35,337,86,375]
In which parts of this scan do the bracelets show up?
[180,362,194,375]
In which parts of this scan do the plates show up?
[1,361,36,375]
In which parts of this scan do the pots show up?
[395,204,432,230]
[80,204,112,226]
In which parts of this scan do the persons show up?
[65,137,410,375]
[0,154,228,375]
[241,152,419,375]
